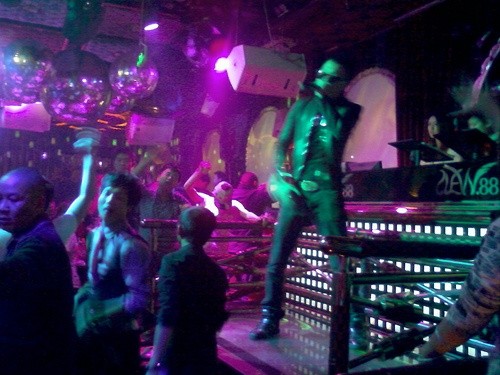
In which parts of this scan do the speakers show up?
[225,45,306,97]
[123,114,175,145]
[0,101,51,133]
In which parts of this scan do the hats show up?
[213,181,233,204]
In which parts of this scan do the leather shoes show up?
[250,317,280,340]
[351,328,370,349]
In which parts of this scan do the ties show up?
[91,231,105,291]
[297,111,322,181]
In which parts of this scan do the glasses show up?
[316,70,344,84]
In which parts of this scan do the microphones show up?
[299,82,319,92]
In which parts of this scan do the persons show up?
[0,168,76,375]
[147,207,227,375]
[417,219,500,375]
[74,171,154,375]
[468,109,491,135]
[0,149,273,341]
[422,112,460,165]
[250,59,369,351]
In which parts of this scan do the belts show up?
[296,179,333,191]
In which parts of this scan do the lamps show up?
[0,1,159,127]
[144,0,159,32]
